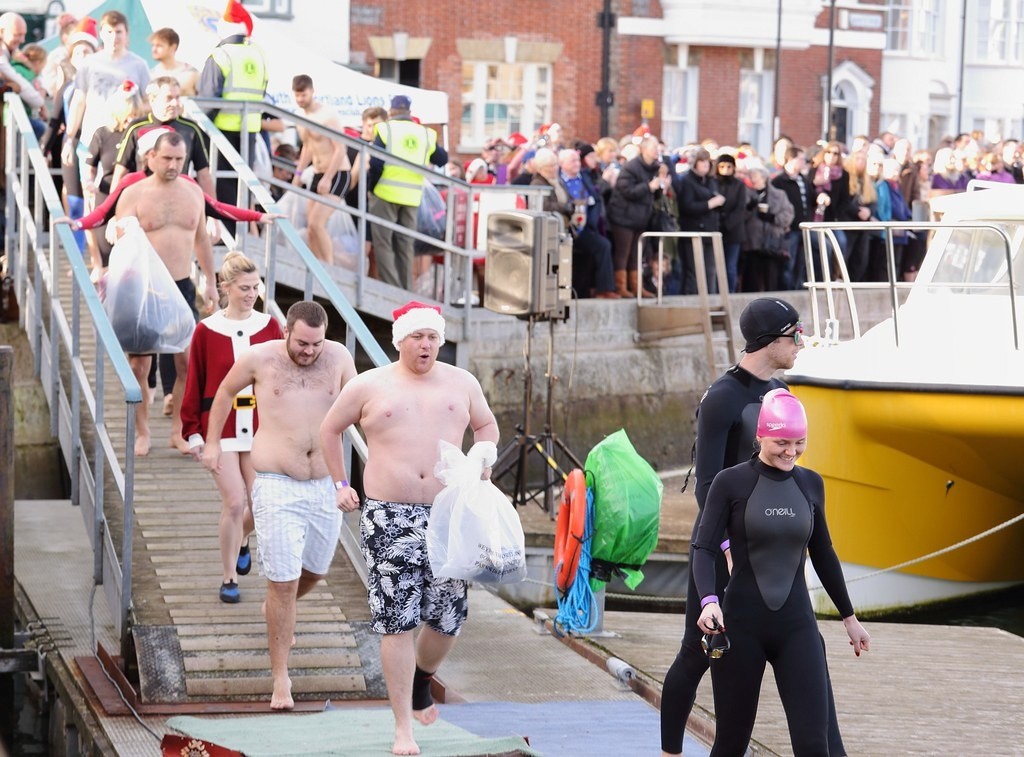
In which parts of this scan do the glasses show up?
[825,150,840,156]
[756,325,802,344]
[700,621,731,659]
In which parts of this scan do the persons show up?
[661,297,805,757]
[0,0,1024,601]
[692,387,872,757]
[202,301,360,710]
[321,300,499,755]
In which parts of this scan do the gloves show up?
[746,195,759,211]
[757,207,775,225]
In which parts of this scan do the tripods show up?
[490,316,569,511]
[491,319,585,522]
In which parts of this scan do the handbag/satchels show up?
[647,207,681,237]
[424,439,529,583]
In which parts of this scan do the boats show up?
[781,177,1024,620]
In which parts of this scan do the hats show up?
[690,148,712,172]
[69,15,99,49]
[391,95,411,109]
[391,301,447,350]
[737,298,800,352]
[137,123,174,158]
[217,0,254,38]
[464,159,488,182]
[755,389,812,438]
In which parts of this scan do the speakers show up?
[515,232,574,324]
[482,208,565,316]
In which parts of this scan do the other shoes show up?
[219,579,241,604]
[237,540,253,575]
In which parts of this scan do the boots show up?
[629,269,655,296]
[615,271,632,298]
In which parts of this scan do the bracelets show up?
[701,596,719,609]
[720,539,730,552]
[334,480,349,490]
